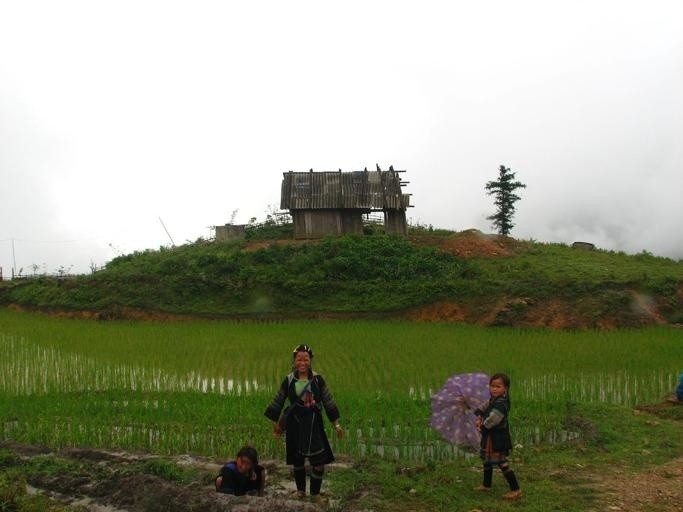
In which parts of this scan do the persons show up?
[264,344,344,502]
[215,446,265,497]
[472,374,523,499]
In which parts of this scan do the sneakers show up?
[290,491,321,503]
[472,485,492,492]
[502,489,522,499]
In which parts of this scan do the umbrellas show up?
[431,372,491,446]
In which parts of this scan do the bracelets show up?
[335,424,340,428]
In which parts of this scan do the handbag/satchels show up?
[278,406,291,424]
